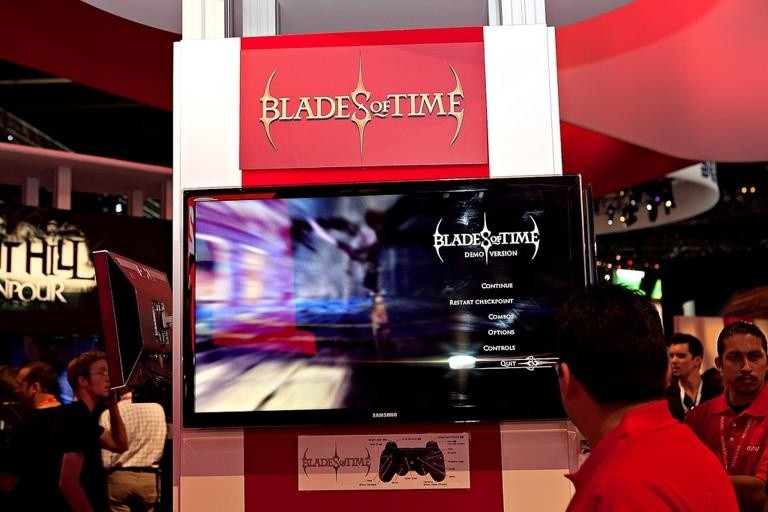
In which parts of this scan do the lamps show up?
[603,179,675,227]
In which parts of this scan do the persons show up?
[98,384,168,511]
[680,321,767,511]
[49,349,129,511]
[1,362,78,510]
[662,331,716,421]
[551,278,740,511]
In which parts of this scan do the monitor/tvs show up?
[180,169,589,425]
[91,247,173,393]
